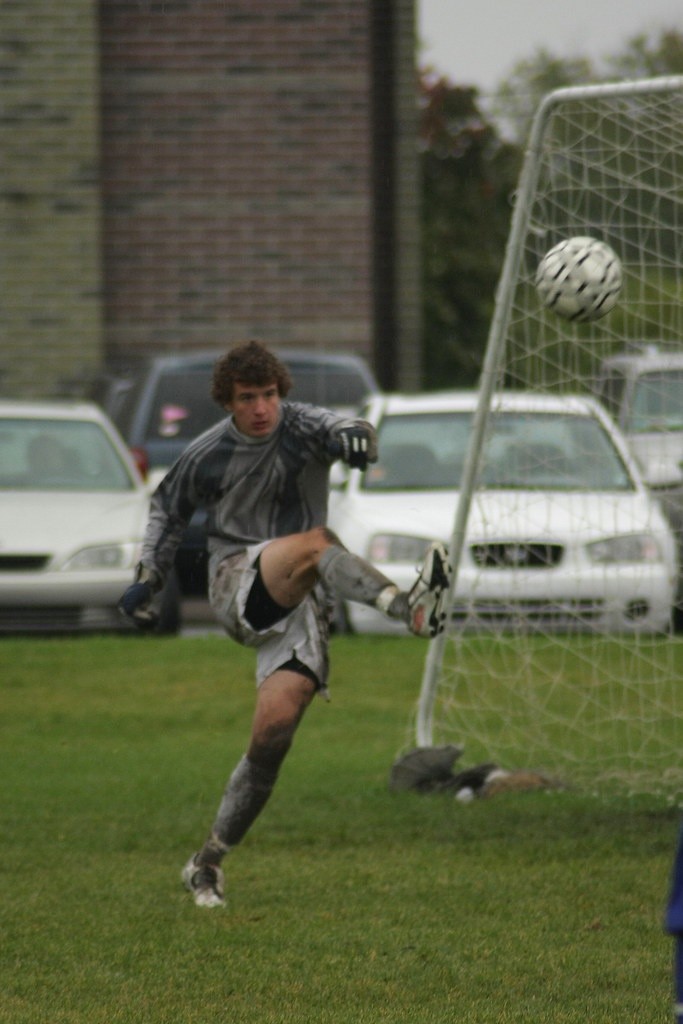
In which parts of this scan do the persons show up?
[118,339,453,908]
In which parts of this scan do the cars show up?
[106,348,383,598]
[310,392,683,639]
[0,394,173,635]
[594,346,682,534]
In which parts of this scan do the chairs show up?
[495,444,573,483]
[380,445,452,486]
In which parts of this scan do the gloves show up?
[327,427,373,472]
[116,583,154,623]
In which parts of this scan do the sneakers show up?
[406,543,455,637]
[181,854,227,910]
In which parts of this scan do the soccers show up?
[536,237,624,322]
